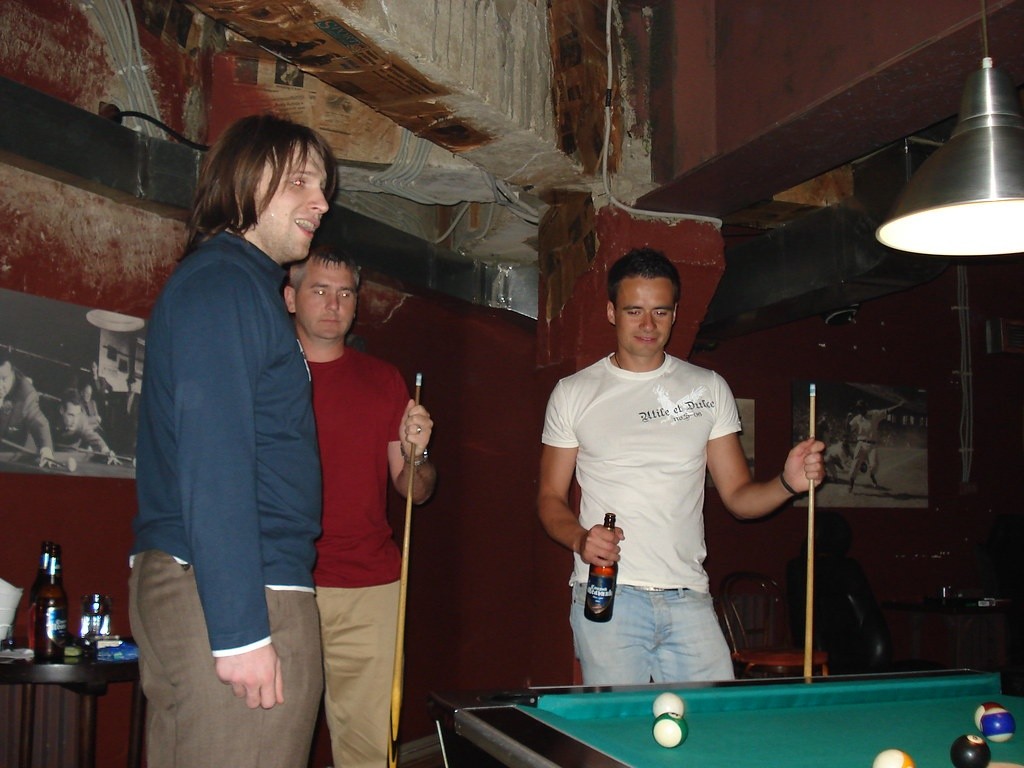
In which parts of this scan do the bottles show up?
[27,542,52,652]
[38,543,66,661]
[78,593,111,640]
[584,512,617,622]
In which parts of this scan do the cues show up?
[803,381,817,682]
[385,370,423,768]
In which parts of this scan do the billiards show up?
[950,734,991,768]
[652,690,689,749]
[871,748,915,768]
[974,701,1016,743]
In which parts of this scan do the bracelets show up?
[779,473,800,497]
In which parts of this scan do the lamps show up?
[874,0,1024,257]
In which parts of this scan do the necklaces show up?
[614,351,622,370]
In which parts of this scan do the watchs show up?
[399,442,428,465]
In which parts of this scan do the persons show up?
[816,414,830,454]
[538,246,829,690]
[278,238,439,768]
[80,379,103,450]
[45,386,123,467]
[90,358,113,420]
[843,398,908,494]
[824,440,855,484]
[124,374,142,456]
[129,112,325,768]
[0,353,57,468]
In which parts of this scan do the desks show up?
[419,665,1024,768]
[879,590,1024,669]
[0,637,147,768]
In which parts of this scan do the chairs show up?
[719,571,829,680]
[783,511,946,676]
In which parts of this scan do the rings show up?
[417,426,422,432]
